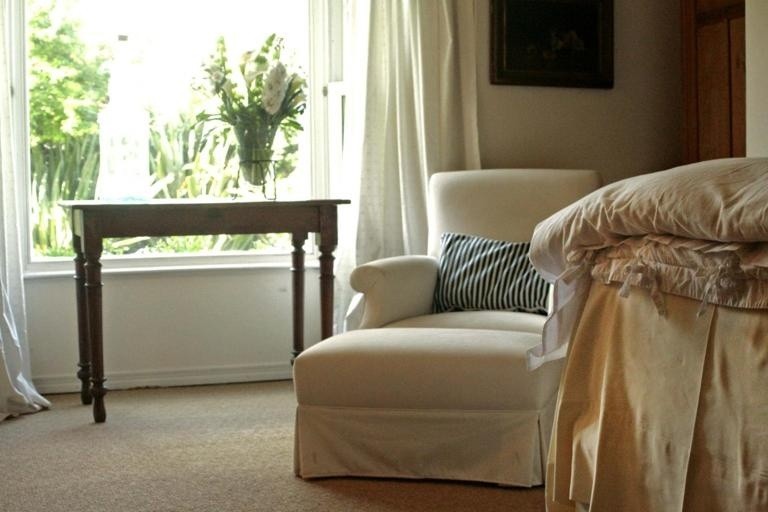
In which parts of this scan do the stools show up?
[291,325,566,487]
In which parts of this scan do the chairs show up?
[346,168,605,338]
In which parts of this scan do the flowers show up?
[195,33,309,188]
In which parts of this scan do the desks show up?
[57,198,351,423]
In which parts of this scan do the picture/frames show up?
[488,1,615,91]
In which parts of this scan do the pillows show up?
[432,230,552,320]
